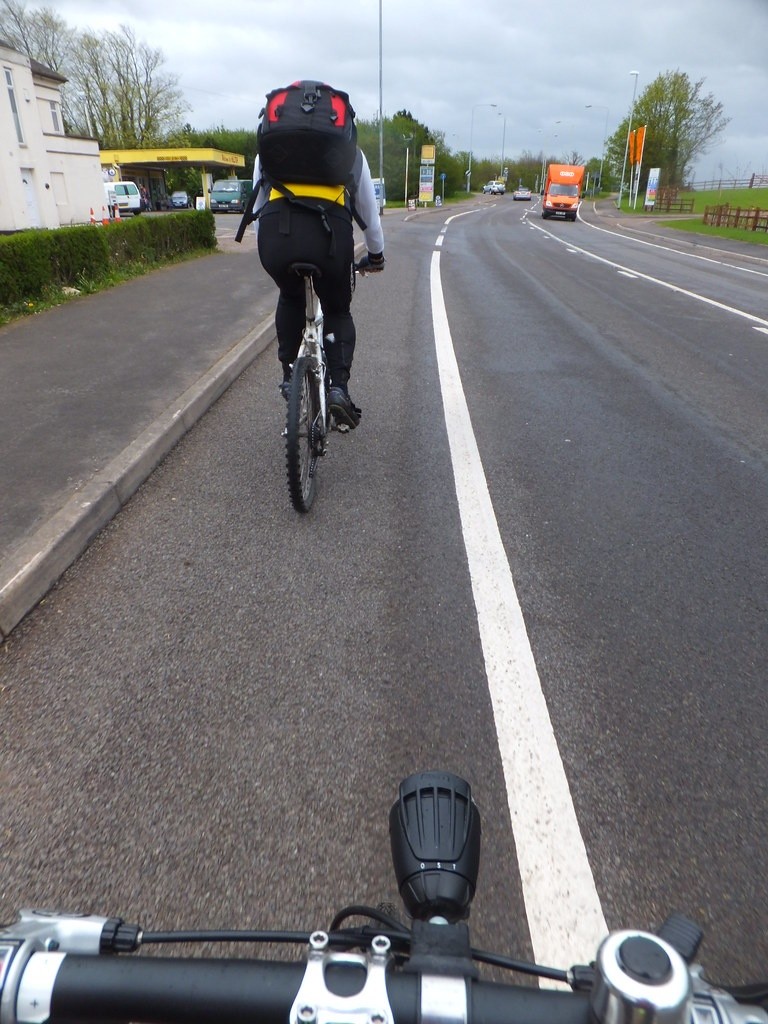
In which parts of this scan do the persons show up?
[253,146,384,430]
[139,184,151,211]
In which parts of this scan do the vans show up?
[105,181,143,218]
[208,179,253,214]
[170,191,190,209]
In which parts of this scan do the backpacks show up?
[256,80,356,186]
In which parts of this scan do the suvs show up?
[482,180,506,195]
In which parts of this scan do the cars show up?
[512,186,532,201]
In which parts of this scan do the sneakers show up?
[327,387,359,430]
[277,382,291,400]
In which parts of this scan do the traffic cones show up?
[113,202,123,222]
[89,208,98,226]
[101,205,110,226]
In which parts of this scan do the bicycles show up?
[279,260,383,514]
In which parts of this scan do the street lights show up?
[616,70,640,209]
[467,104,498,193]
[586,104,609,188]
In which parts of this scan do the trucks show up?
[541,163,585,222]
